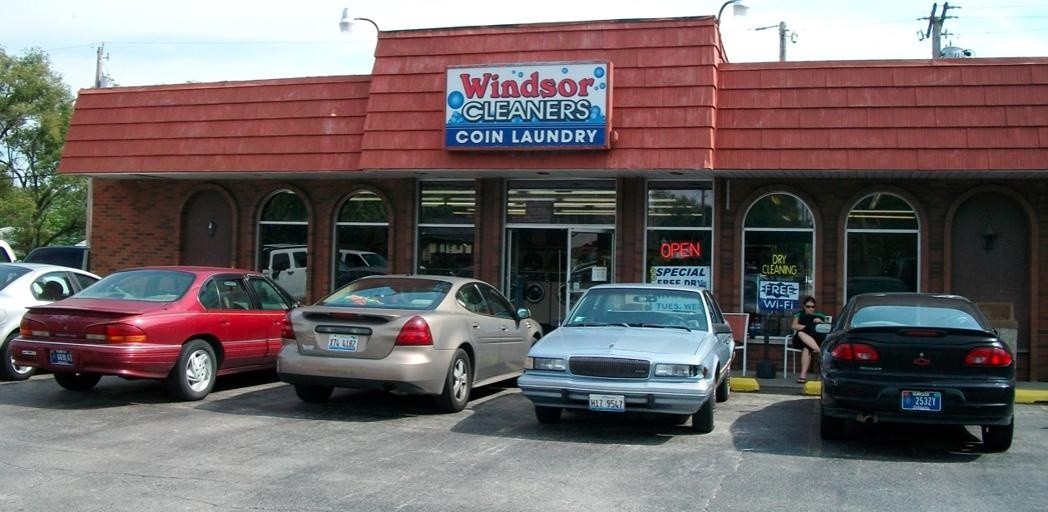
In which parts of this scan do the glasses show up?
[805,306,815,308]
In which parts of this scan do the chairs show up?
[718,310,831,382]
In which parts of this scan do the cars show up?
[12,264,294,402]
[816,290,1016,457]
[0,239,131,387]
[517,281,737,436]
[274,273,543,414]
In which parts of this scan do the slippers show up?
[796,377,806,383]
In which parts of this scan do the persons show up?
[788,296,829,384]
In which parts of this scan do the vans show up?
[264,243,387,298]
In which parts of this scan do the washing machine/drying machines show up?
[519,269,578,325]
